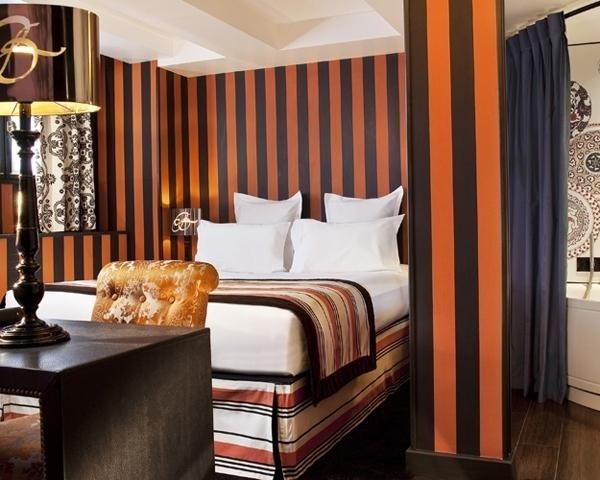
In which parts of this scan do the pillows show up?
[193,185,406,275]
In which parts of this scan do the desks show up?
[0,317,216,480]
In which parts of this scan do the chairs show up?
[0,259,220,480]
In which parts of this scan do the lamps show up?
[168,207,201,263]
[0,96,103,348]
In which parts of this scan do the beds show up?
[4,260,411,480]
